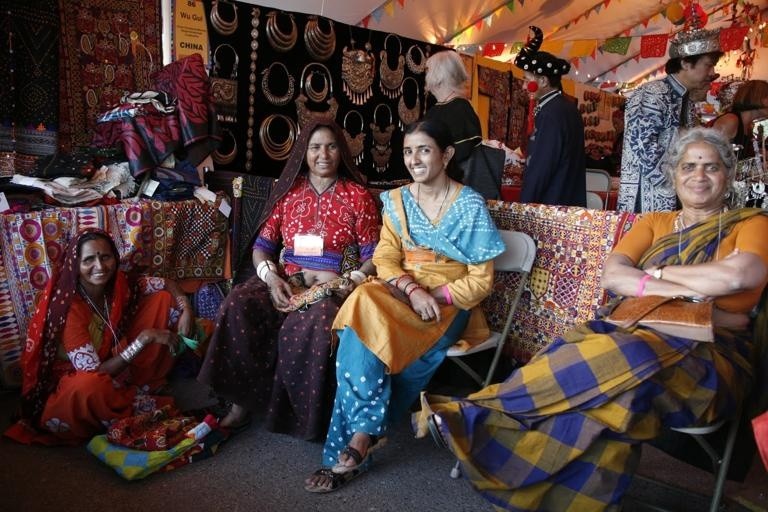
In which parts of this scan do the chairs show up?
[429,230,538,480]
[581,168,613,213]
[654,291,767,511]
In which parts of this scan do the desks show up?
[1,194,231,392]
[499,183,523,203]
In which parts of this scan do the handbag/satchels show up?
[459,142,507,201]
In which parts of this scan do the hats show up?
[668,24,723,61]
[512,49,571,75]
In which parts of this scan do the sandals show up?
[426,413,449,451]
[303,464,370,494]
[212,414,250,438]
[331,432,389,474]
